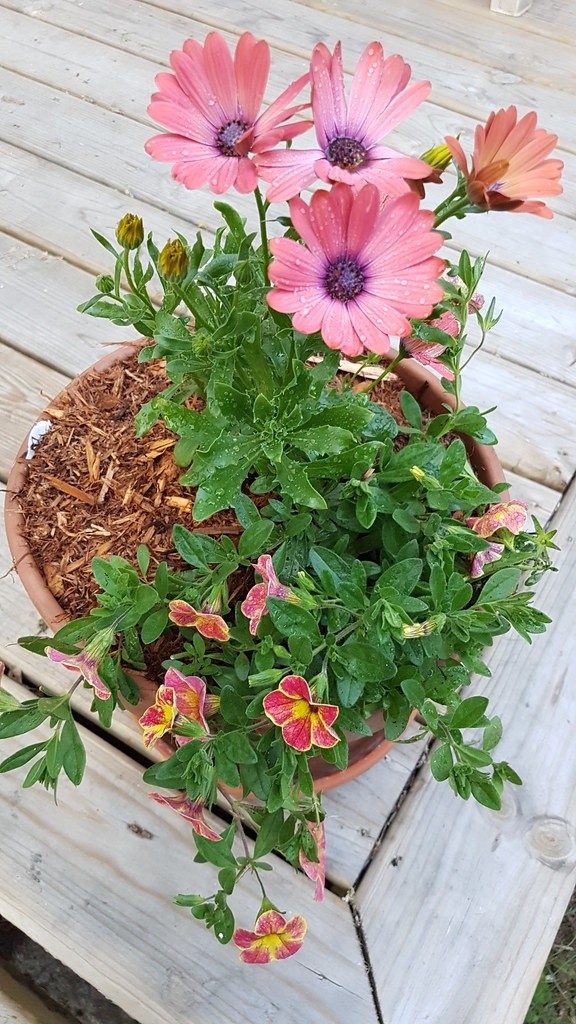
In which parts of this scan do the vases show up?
[6,335,504,805]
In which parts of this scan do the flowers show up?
[0,29,575,968]
